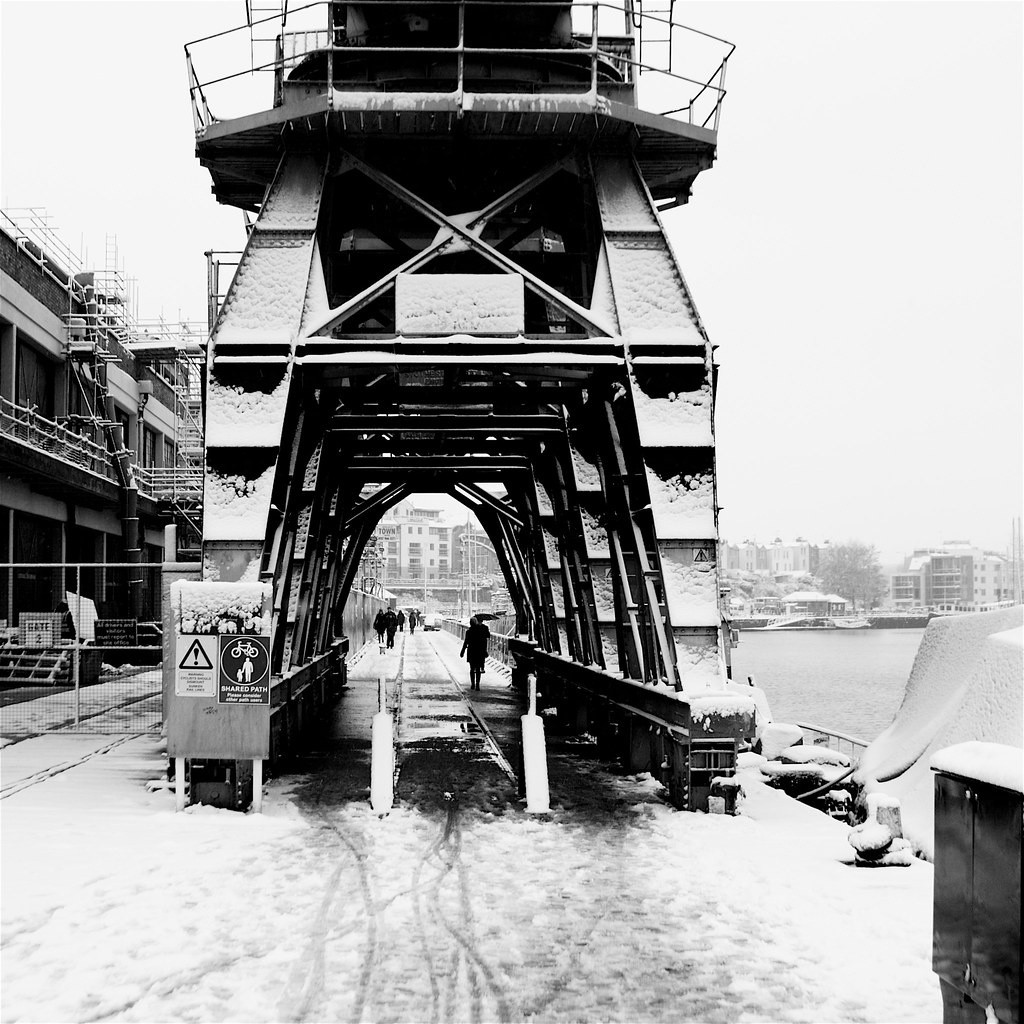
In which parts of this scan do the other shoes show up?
[474,668,476,673]
[476,683,480,691]
[390,645,393,649]
[471,684,475,690]
[480,669,485,673]
[387,645,389,649]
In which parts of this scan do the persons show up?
[373,607,421,650]
[457,615,492,692]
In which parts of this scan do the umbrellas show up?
[474,613,500,620]
